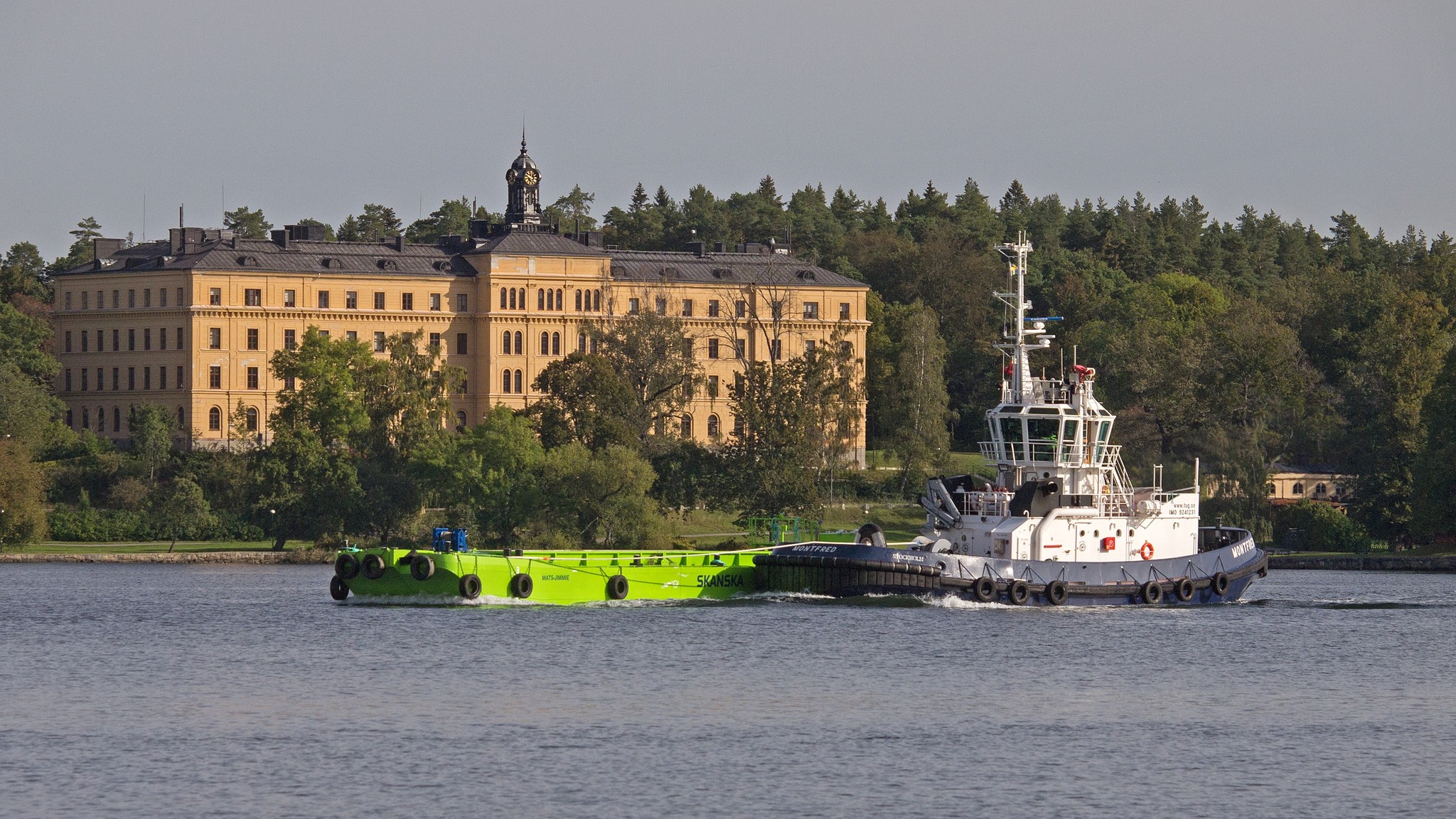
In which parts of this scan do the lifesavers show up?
[1212,572,1230,596]
[608,574,629,600]
[973,576,997,602]
[1175,578,1195,602]
[410,555,434,581]
[1142,581,1163,605]
[1008,580,1030,606]
[1047,580,1068,605]
[330,575,349,601]
[334,553,360,580]
[361,553,385,579]
[459,574,482,600]
[1141,542,1154,560]
[511,573,533,598]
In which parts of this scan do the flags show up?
[1010,261,1025,276]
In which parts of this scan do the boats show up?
[753,229,1268,606]
[329,518,922,608]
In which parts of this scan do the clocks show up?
[506,170,515,185]
[524,169,539,185]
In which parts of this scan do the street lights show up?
[270,510,276,548]
